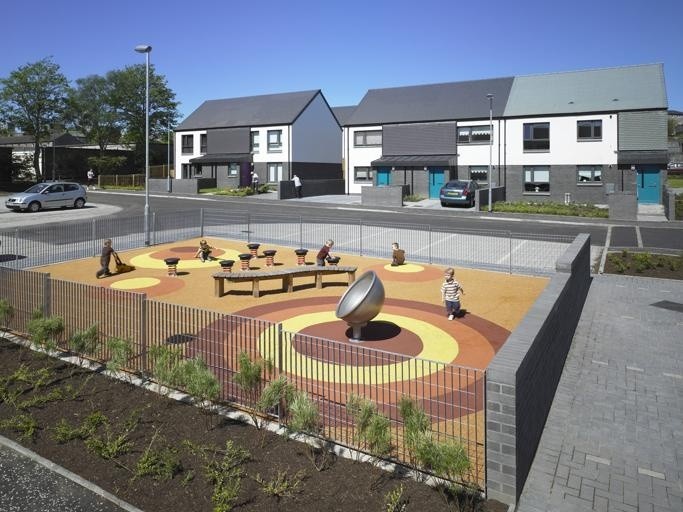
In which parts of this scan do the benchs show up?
[211,265,357,297]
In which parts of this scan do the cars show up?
[440,180,479,207]
[5,182,87,212]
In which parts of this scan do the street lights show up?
[135,44,152,244]
[486,94,494,208]
[163,111,178,192]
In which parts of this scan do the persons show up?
[466,174,479,206]
[94,238,116,279]
[289,174,303,198]
[250,169,260,194]
[86,168,97,191]
[193,239,215,262]
[389,241,404,266]
[313,238,334,265]
[439,267,464,321]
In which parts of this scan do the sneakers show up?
[95,271,111,278]
[448,314,454,321]
